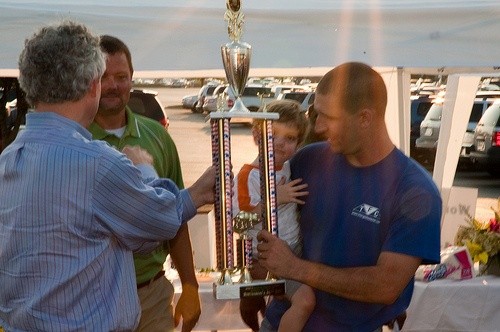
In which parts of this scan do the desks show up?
[169,266,254,331]
[399,270,500,331]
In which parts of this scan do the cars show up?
[129,76,192,89]
[475,100,500,176]
[182,75,500,148]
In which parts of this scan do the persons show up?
[84,34,202,331]
[237,98,319,332]
[254,60,445,332]
[1,19,237,332]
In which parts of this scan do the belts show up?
[137,269,165,290]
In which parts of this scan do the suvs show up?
[415,100,493,154]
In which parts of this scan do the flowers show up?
[455,206,500,264]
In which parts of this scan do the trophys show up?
[206,1,289,302]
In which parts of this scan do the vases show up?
[478,254,499,276]
